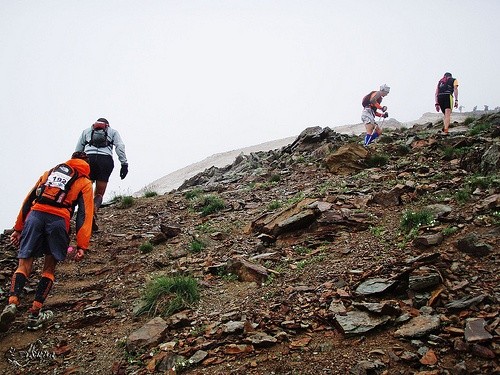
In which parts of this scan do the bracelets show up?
[435,104,440,106]
[455,100,458,102]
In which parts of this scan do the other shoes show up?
[92,214,99,231]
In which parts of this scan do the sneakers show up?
[27,310,54,330]
[0,303,16,332]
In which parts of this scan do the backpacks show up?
[362,91,376,106]
[91,121,109,148]
[438,75,449,93]
[36,163,78,208]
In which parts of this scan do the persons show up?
[72,117,129,229]
[0,151,95,334]
[434,72,459,133]
[361,83,390,146]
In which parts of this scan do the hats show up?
[380,86,390,92]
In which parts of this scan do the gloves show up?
[385,112,388,117]
[382,106,387,112]
[120,163,128,179]
